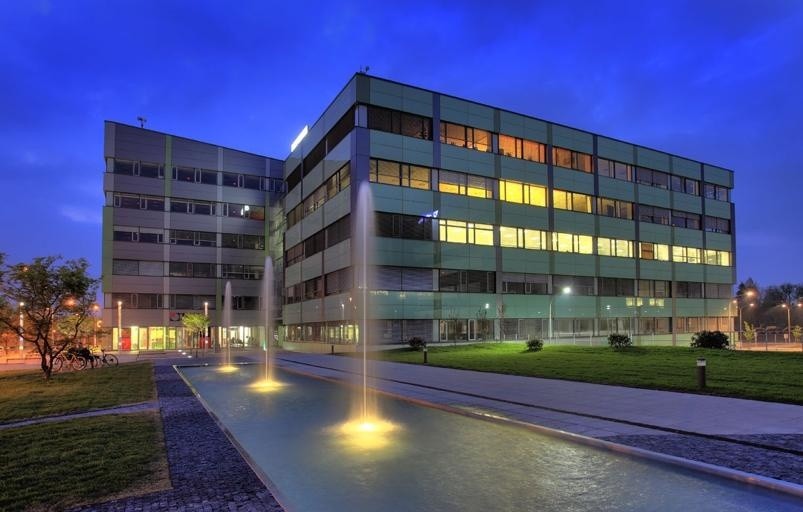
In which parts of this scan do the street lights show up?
[548,287,569,341]
[727,291,754,351]
[781,302,802,344]
[19,300,24,357]
[484,303,490,341]
[117,300,122,351]
[203,301,208,354]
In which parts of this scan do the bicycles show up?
[47,344,119,372]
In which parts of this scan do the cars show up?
[737,325,802,339]
